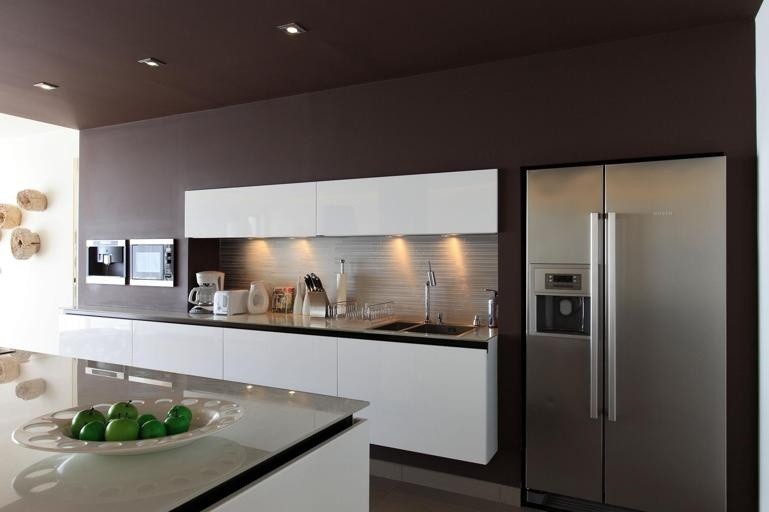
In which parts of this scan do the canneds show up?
[272,287,295,313]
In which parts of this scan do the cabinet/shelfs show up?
[223,325,498,470]
[182,167,502,240]
[60,314,225,386]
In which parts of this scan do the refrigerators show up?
[519,156,750,512]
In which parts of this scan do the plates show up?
[11,396,244,456]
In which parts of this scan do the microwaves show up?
[132,245,175,281]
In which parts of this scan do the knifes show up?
[303,273,324,292]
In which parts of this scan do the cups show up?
[361,299,395,323]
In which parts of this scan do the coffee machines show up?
[188,270,225,314]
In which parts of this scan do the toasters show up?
[214,289,250,316]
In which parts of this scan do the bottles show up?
[293,275,311,317]
[335,259,347,317]
[246,280,269,315]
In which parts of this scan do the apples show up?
[69,398,192,441]
